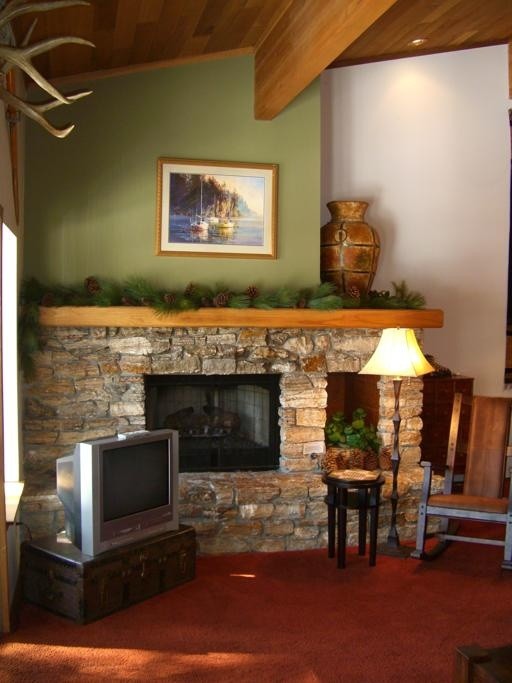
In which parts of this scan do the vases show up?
[320,200,381,300]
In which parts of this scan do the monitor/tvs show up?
[55,428,179,556]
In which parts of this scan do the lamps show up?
[357,326,437,560]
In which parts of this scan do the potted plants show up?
[323,407,382,484]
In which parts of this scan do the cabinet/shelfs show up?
[20,524,195,626]
[422,375,475,495]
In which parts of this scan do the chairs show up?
[411,392,512,571]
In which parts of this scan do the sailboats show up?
[190,229,209,243]
[189,178,237,231]
[216,228,234,241]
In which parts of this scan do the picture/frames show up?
[156,157,279,260]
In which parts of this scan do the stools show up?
[455,645,512,682]
[323,468,386,569]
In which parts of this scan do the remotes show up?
[117,429,151,440]
